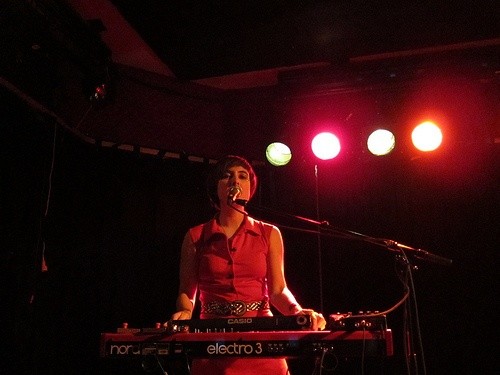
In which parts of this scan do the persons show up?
[170,156,326,375]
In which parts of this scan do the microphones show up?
[227,186,243,207]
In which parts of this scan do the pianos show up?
[96,311,395,363]
